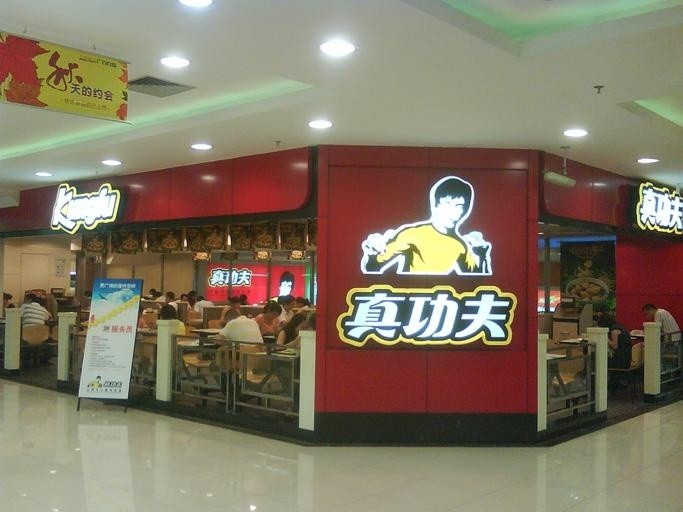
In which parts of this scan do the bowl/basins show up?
[566,277,609,303]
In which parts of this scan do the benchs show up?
[81,294,316,328]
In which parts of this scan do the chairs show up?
[21,313,300,409]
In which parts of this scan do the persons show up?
[3,292,51,368]
[221,294,312,335]
[270,312,316,397]
[641,302,682,360]
[575,312,633,384]
[359,175,495,279]
[150,289,214,315]
[138,305,178,382]
[216,310,263,397]
